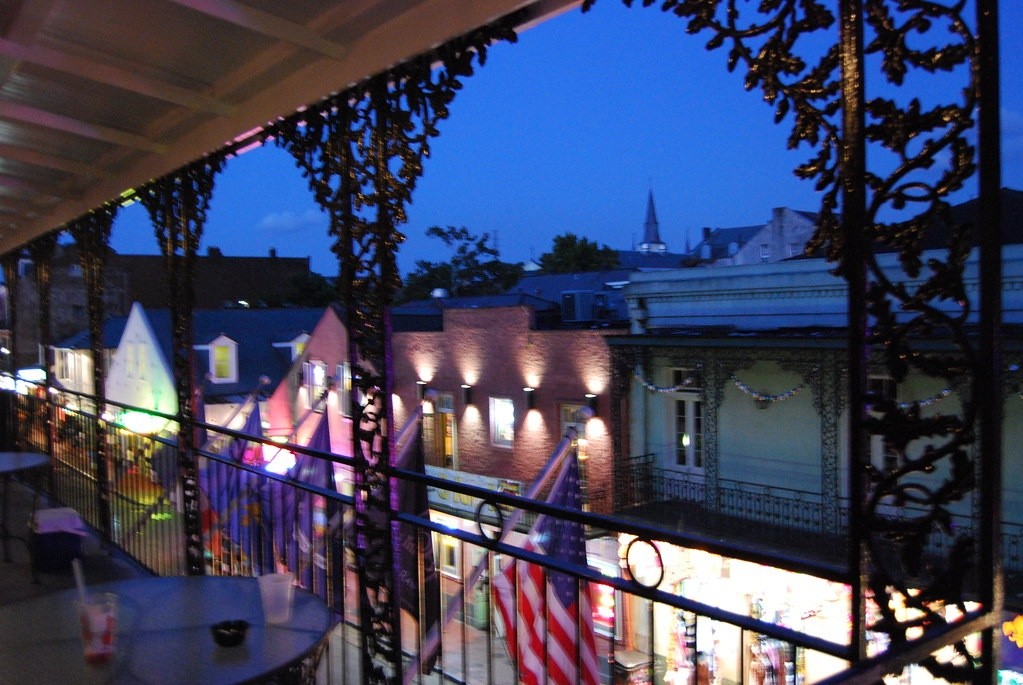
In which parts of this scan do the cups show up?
[77,591,117,666]
[258,574,291,624]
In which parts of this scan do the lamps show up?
[524,387,535,409]
[585,394,597,417]
[417,380,426,400]
[460,384,471,405]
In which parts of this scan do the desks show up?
[0,576,330,685]
[0,451,50,560]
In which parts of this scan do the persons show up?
[113,444,159,483]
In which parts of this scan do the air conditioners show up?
[559,289,595,322]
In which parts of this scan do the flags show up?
[196,402,266,575]
[151,404,221,557]
[340,428,442,675]
[485,450,600,685]
[253,407,343,621]
